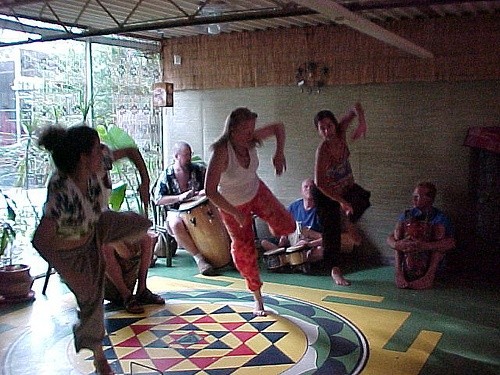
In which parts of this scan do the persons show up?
[310,101,371,286]
[154,141,213,275]
[103,235,166,314]
[261,178,364,271]
[32,124,150,375]
[206,107,296,317]
[385,182,456,290]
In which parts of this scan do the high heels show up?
[93,356,114,375]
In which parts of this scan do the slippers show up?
[123,294,145,314]
[135,288,166,304]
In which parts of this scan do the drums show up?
[263,247,286,269]
[286,245,312,267]
[178,189,231,269]
[402,220,432,279]
[103,244,140,301]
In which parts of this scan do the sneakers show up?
[192,253,214,275]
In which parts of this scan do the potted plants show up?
[0,188,35,298]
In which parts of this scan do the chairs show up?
[150,178,174,266]
